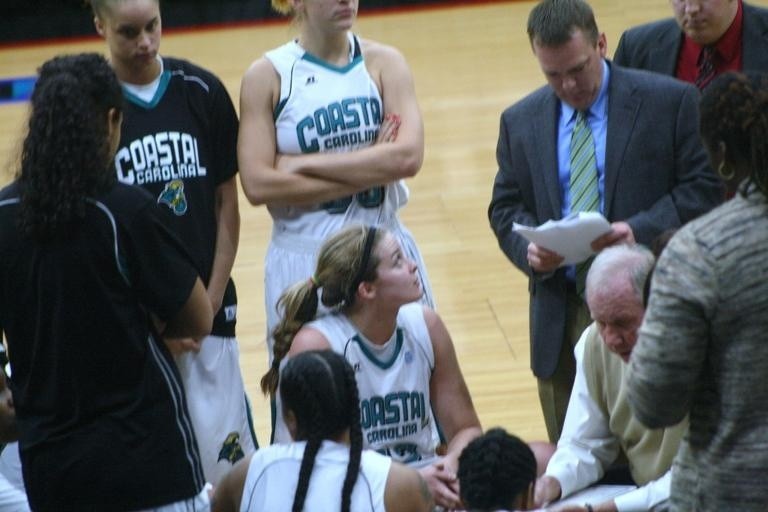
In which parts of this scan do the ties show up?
[568,110,601,303]
[694,43,723,95]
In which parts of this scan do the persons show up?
[628,69,768,512]
[238,0,435,444]
[488,0,729,447]
[92,0,260,491]
[613,1,768,202]
[458,426,537,512]
[262,222,486,512]
[211,349,435,512]
[534,244,688,512]
[0,53,214,512]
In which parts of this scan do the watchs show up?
[585,503,592,512]
